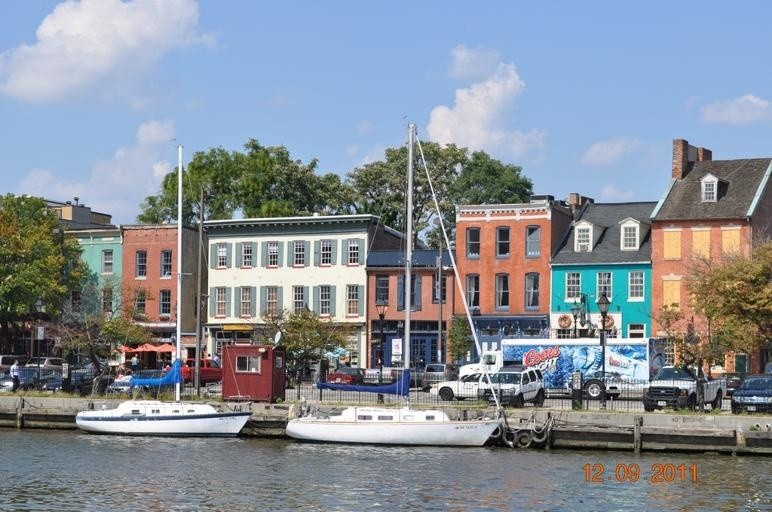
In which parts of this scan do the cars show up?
[283,361,303,389]
[719,371,744,396]
[0,351,223,394]
[728,374,772,415]
[325,363,549,407]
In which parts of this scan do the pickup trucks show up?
[640,362,728,412]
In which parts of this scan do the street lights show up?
[596,287,615,410]
[34,294,50,392]
[570,298,580,338]
[376,292,390,406]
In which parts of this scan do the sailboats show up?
[281,120,512,449]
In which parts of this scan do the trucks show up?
[456,337,655,402]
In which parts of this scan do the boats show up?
[71,139,259,441]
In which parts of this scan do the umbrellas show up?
[110,344,132,356]
[129,342,158,353]
[154,343,177,355]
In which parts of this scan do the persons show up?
[10,359,22,394]
[207,351,212,360]
[130,353,140,374]
[213,351,220,366]
